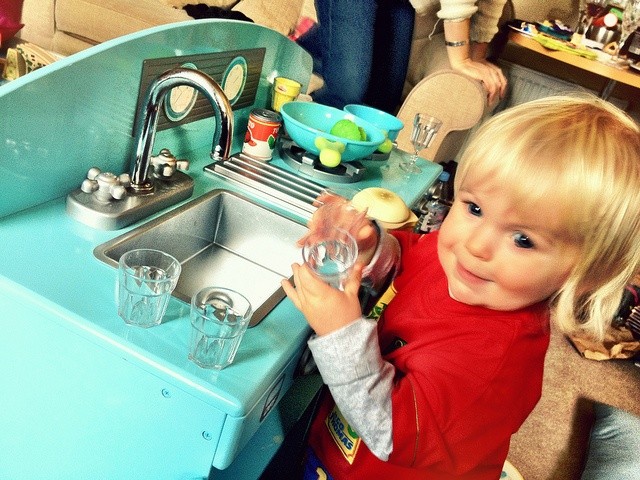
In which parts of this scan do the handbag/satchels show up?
[444,40,470,48]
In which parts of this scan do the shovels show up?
[351,184,411,224]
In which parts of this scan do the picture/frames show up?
[510,19,640,100]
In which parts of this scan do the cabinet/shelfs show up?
[418,171,449,228]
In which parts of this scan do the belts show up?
[399,113,443,172]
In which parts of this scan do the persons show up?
[281,94,640,480]
[314,0,509,116]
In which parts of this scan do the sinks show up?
[1,0,500,162]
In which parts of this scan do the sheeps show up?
[126,68,233,194]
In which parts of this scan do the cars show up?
[302,228,358,281]
[116,249,181,328]
[188,287,253,369]
